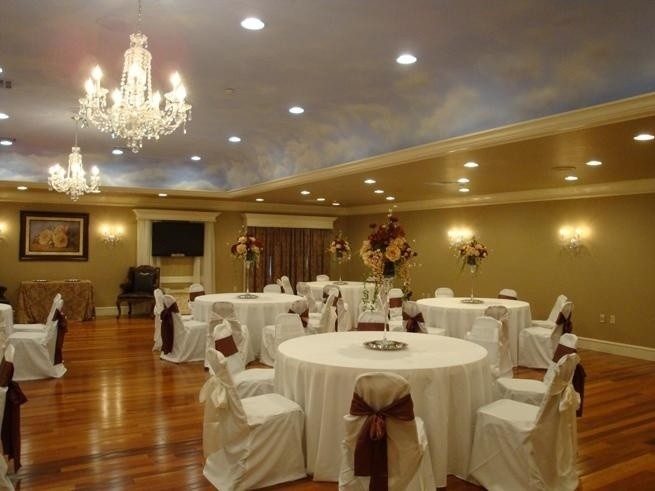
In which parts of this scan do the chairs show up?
[0,344,29,490]
[2,299,67,381]
[12,293,62,332]
[151,273,350,372]
[292,272,568,364]
[200,348,307,489]
[0,286,10,304]
[518,301,572,369]
[116,265,160,319]
[496,333,577,405]
[212,323,276,397]
[341,372,435,488]
[466,353,580,490]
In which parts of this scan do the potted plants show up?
[19,210,90,262]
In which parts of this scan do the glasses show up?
[558,226,590,255]
[74,0,193,149]
[47,116,101,202]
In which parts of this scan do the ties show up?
[151,220,205,258]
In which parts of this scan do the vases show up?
[462,267,483,304]
[241,261,256,299]
[448,236,489,266]
[223,225,264,269]
[324,231,353,265]
[354,205,423,311]
[334,262,348,285]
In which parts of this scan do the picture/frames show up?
[19,210,90,262]
[15,279,96,324]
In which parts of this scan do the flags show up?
[334,262,348,285]
[462,267,483,304]
[241,261,256,299]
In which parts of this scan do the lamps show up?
[47,116,101,202]
[558,226,590,255]
[12,293,62,332]
[2,299,67,381]
[74,0,193,149]
[0,344,29,490]
[0,286,10,304]
[116,265,160,319]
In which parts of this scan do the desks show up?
[15,279,96,324]
[277,331,489,482]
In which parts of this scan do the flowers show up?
[354,205,423,311]
[324,231,353,265]
[223,225,264,269]
[448,236,489,266]
